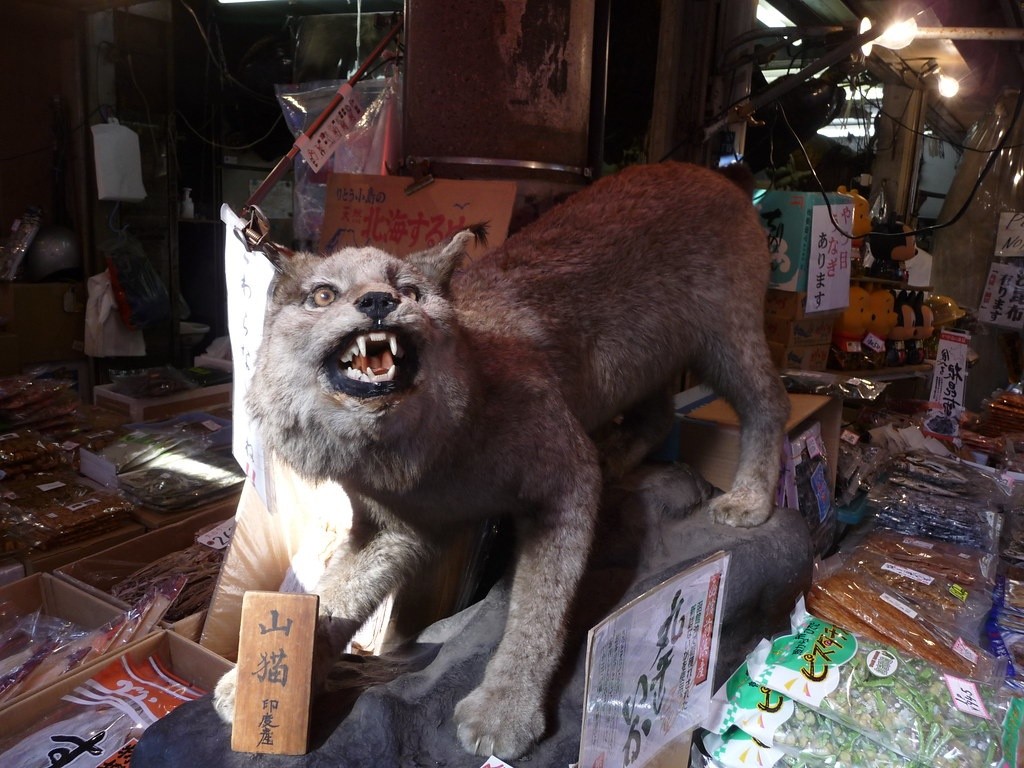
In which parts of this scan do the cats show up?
[211,157,793,764]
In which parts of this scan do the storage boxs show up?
[93,379,232,425]
[0,282,88,396]
[0,456,270,768]
[652,380,844,506]
[752,191,855,371]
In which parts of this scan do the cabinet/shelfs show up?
[835,272,934,525]
[112,125,222,375]
